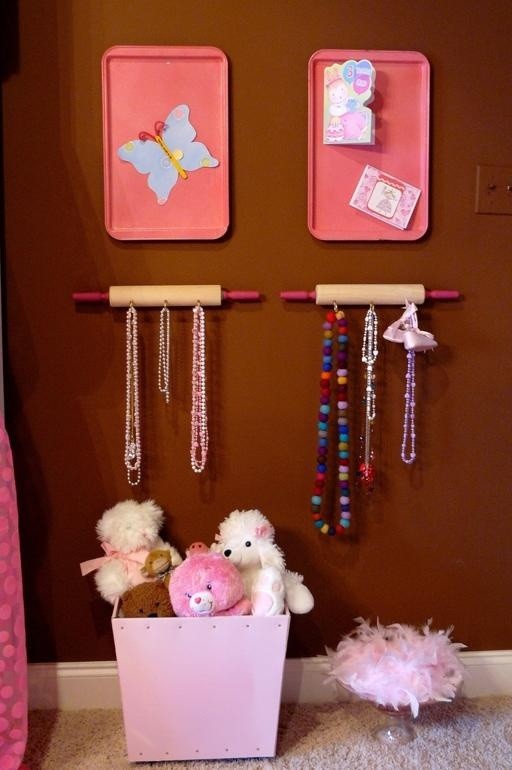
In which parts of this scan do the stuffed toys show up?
[78,497,183,606]
[119,577,177,618]
[185,542,209,557]
[169,552,252,617]
[210,508,315,617]
[140,548,176,588]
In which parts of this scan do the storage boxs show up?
[108,595,293,764]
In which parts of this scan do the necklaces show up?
[190,305,209,473]
[382,298,438,465]
[362,308,379,482]
[124,306,141,485]
[158,307,170,404]
[311,309,351,536]
